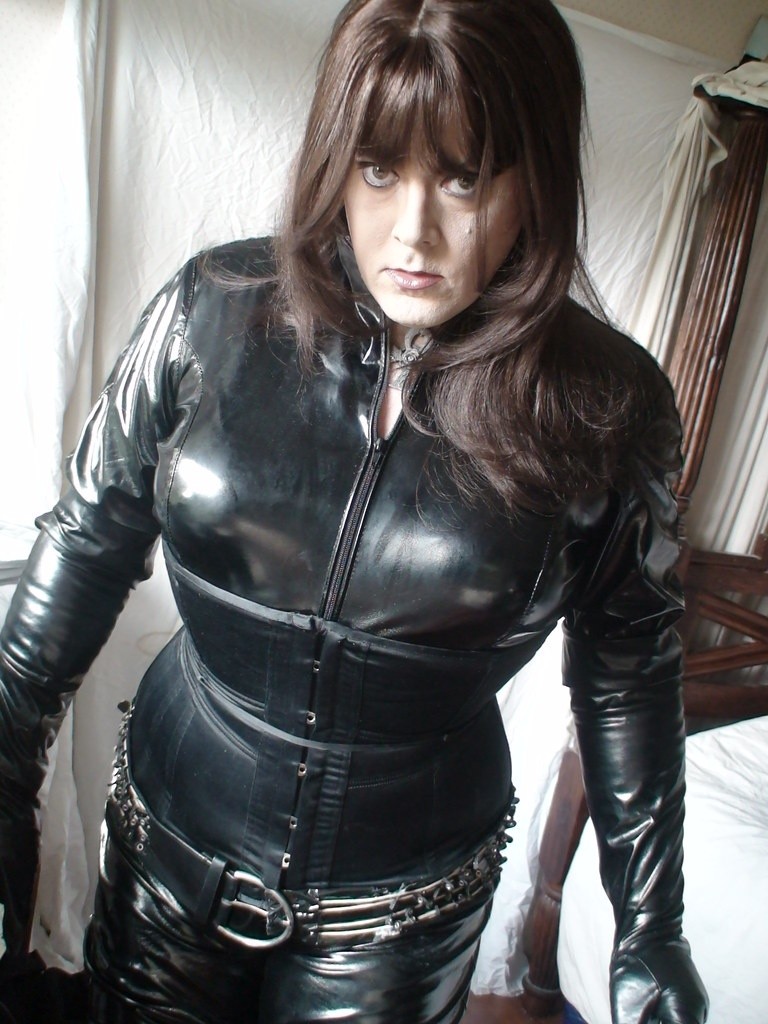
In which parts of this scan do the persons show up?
[0,0,711,1024]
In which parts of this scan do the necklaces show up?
[381,326,435,391]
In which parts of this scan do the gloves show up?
[608,938,711,1024]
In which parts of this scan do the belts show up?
[102,698,520,960]
